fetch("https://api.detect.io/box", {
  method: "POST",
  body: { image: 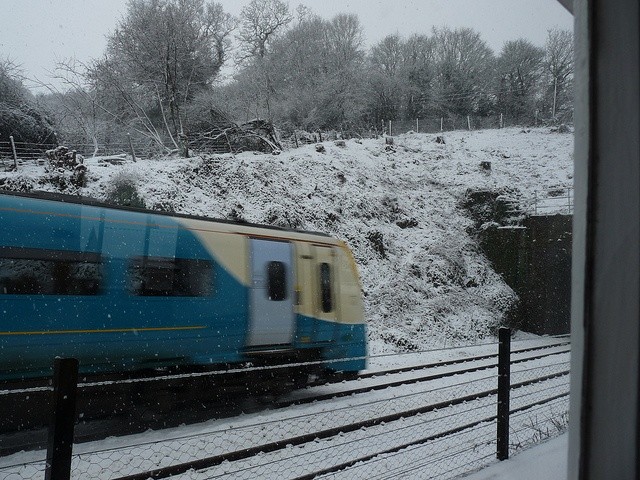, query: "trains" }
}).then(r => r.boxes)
[0,189,366,424]
